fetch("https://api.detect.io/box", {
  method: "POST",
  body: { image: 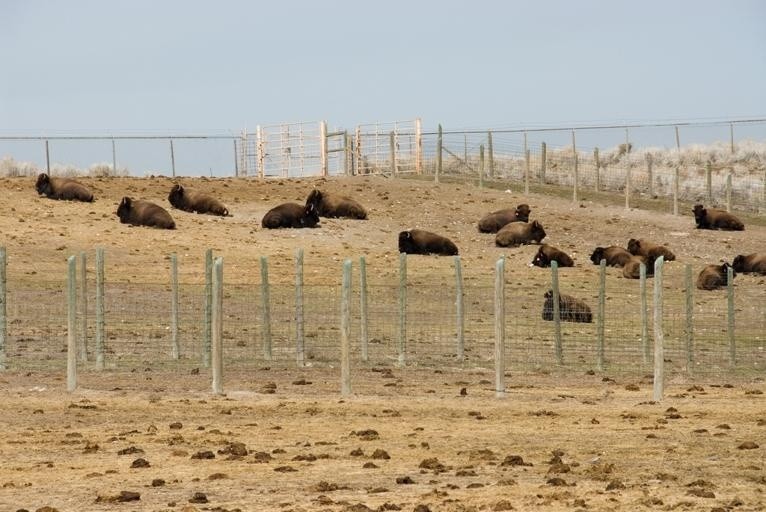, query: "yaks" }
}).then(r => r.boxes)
[168,183,228,216]
[479,205,531,233]
[696,263,736,291]
[732,250,766,276]
[117,197,175,229]
[532,245,574,267]
[262,203,320,229]
[305,185,366,220]
[692,205,744,231]
[496,220,546,248]
[36,174,93,202]
[399,230,458,255]
[542,290,592,323]
[591,239,676,279]
